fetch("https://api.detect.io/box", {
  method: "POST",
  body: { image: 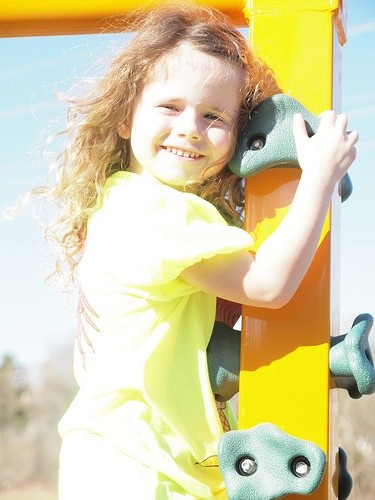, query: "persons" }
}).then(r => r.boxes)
[50,2,360,500]
